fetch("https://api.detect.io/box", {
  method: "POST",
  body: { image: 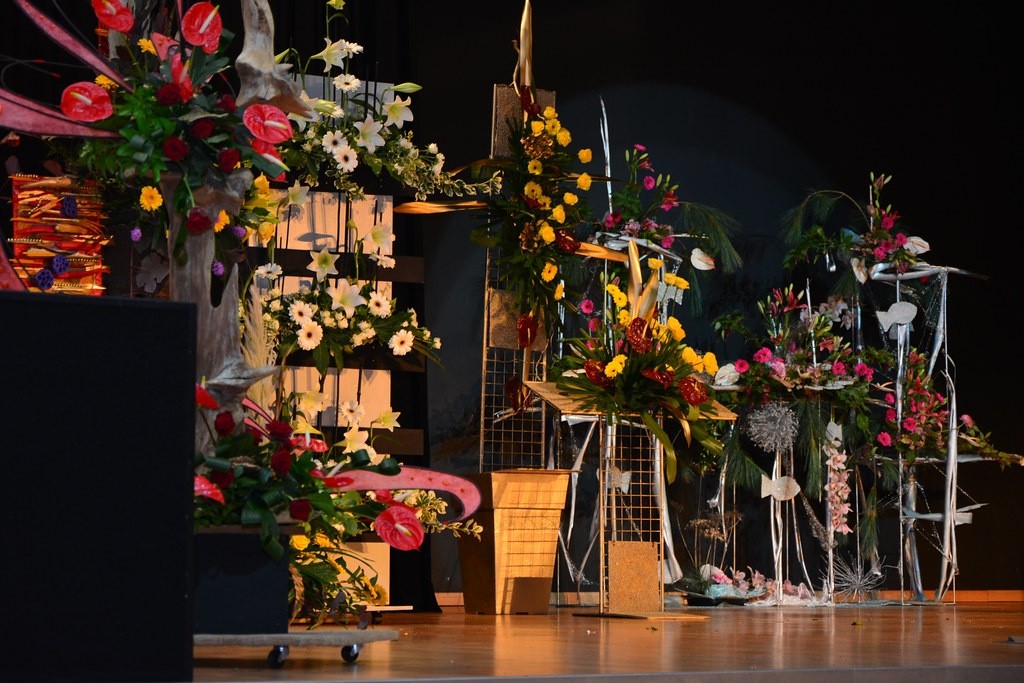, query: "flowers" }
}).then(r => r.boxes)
[0,0,1024,631]
